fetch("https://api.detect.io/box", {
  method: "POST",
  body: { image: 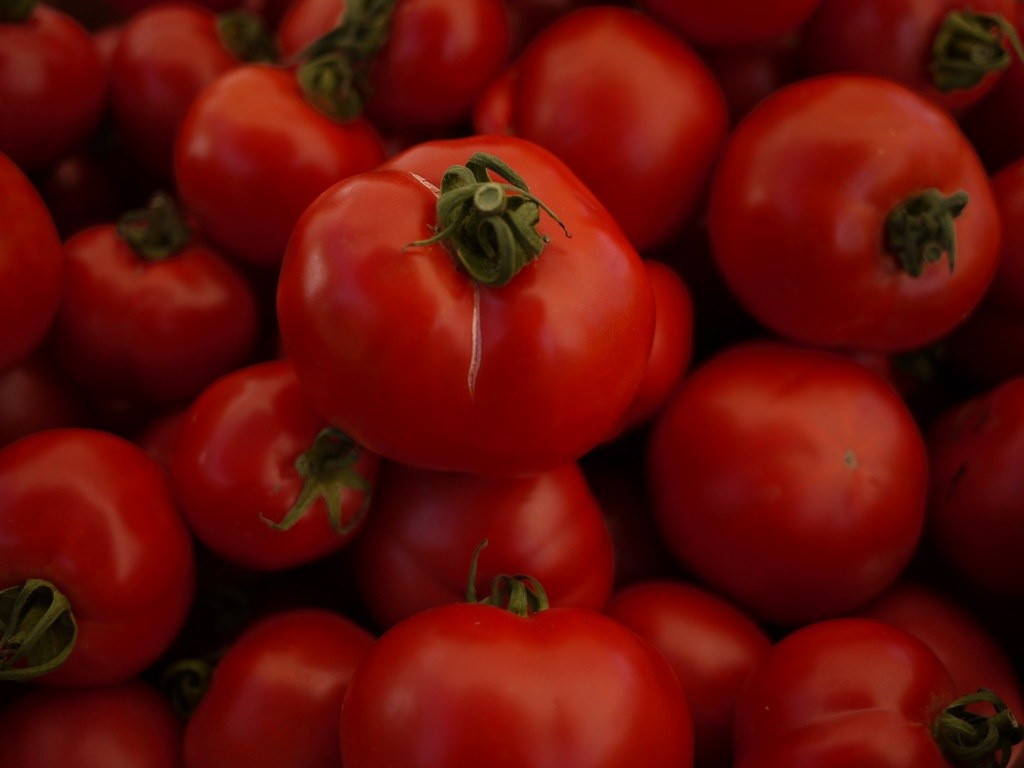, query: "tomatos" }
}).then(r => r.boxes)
[0,0,1024,768]
